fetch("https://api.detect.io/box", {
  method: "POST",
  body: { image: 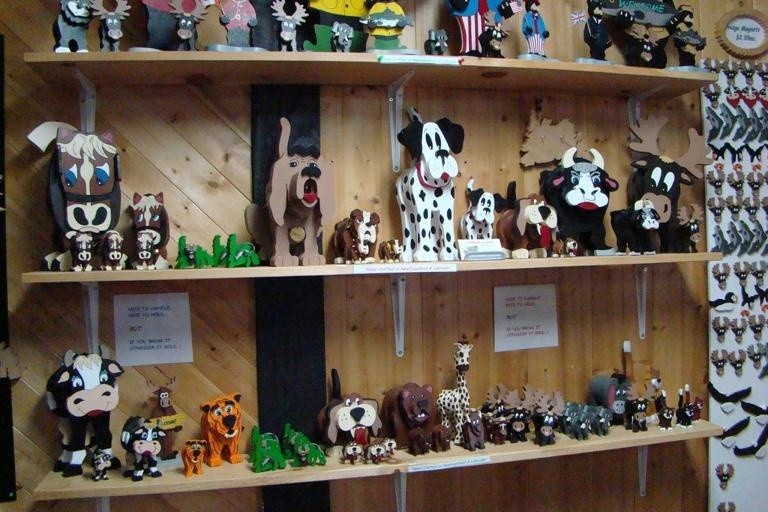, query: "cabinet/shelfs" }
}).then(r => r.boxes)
[19,52,723,510]
